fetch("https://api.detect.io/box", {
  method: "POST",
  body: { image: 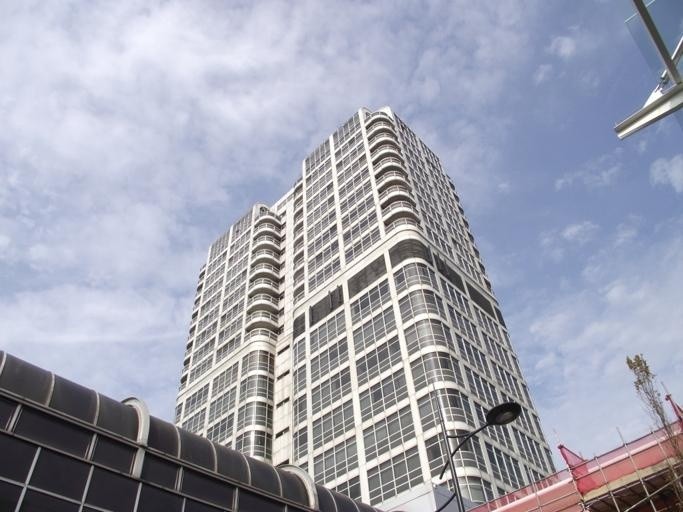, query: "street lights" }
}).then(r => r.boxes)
[438,401,522,511]
